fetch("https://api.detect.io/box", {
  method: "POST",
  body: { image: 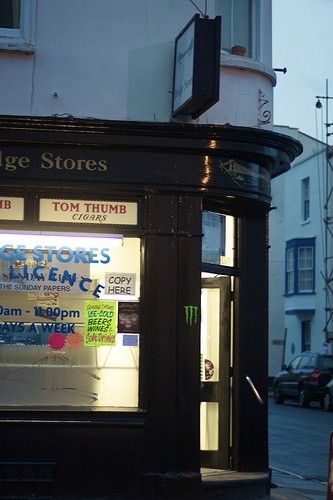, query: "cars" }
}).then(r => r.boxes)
[273,352,333,409]
